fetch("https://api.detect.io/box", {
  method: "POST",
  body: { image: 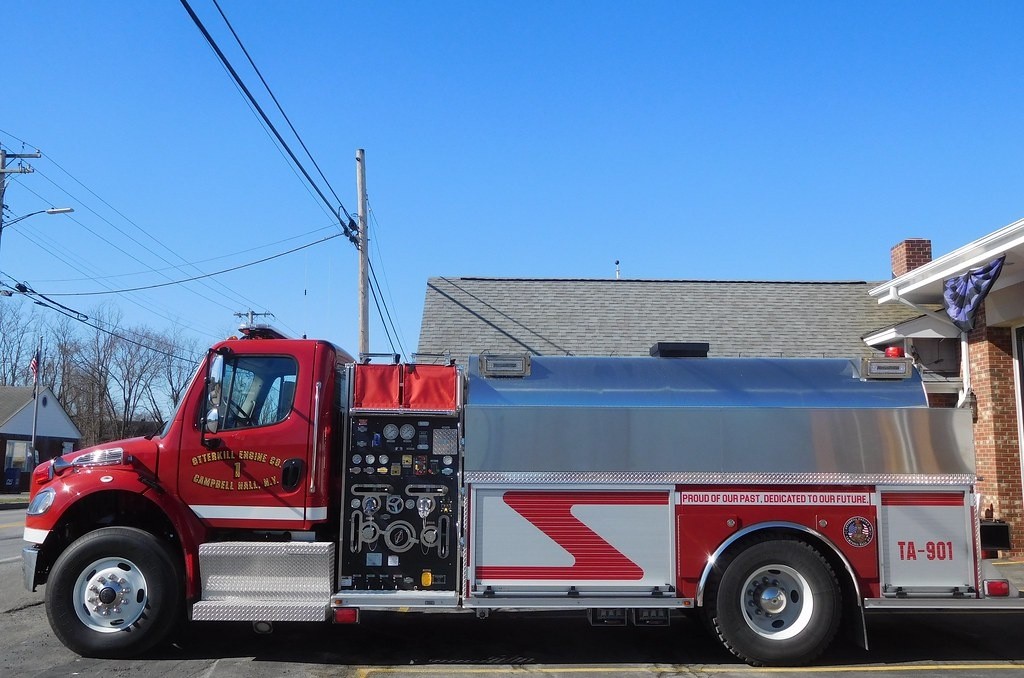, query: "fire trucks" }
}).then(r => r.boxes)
[19,320,1023,668]
